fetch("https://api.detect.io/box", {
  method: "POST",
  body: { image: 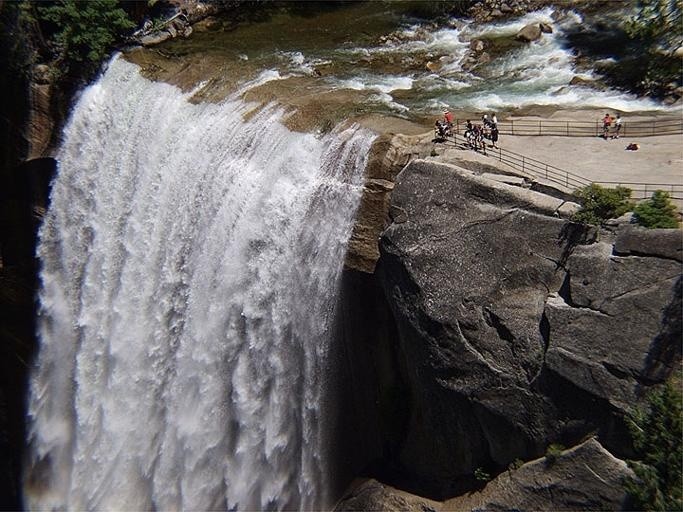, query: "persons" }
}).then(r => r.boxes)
[435,109,498,148]
[613,116,622,138]
[603,114,612,138]
